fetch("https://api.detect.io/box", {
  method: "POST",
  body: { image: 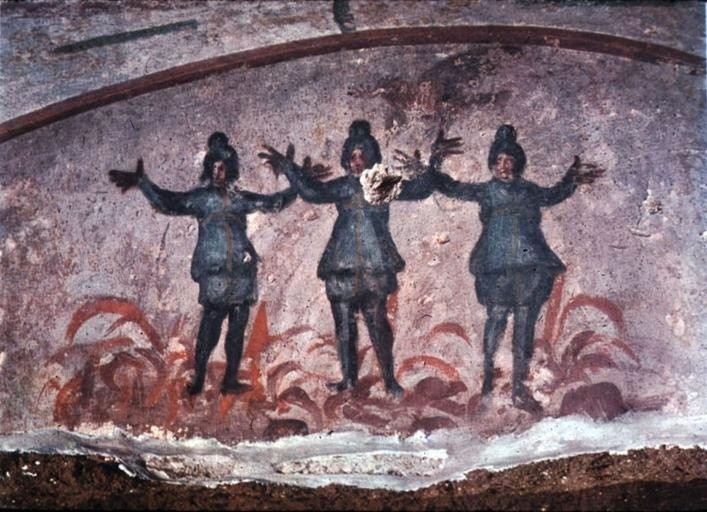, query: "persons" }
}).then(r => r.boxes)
[392,124,606,406]
[257,120,464,395]
[108,132,334,395]
